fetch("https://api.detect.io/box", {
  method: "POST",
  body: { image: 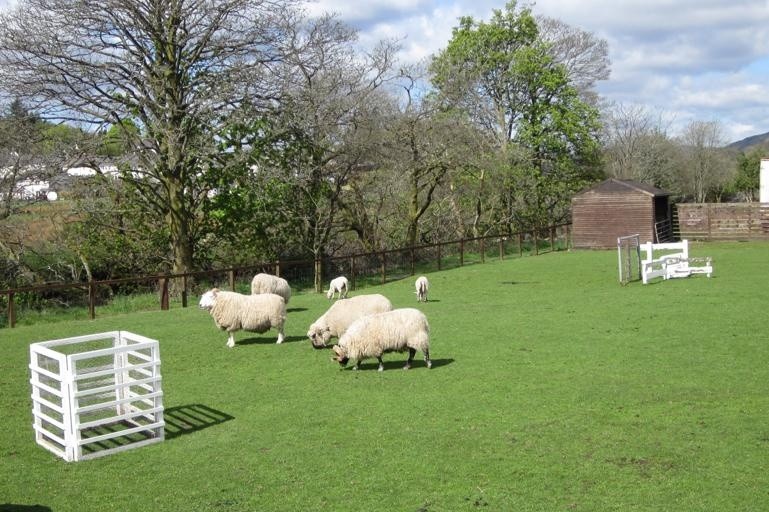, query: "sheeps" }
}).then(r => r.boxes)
[412,277,429,303]
[306,294,393,349]
[199,288,287,348]
[327,276,349,300]
[331,308,432,372]
[251,273,292,304]
